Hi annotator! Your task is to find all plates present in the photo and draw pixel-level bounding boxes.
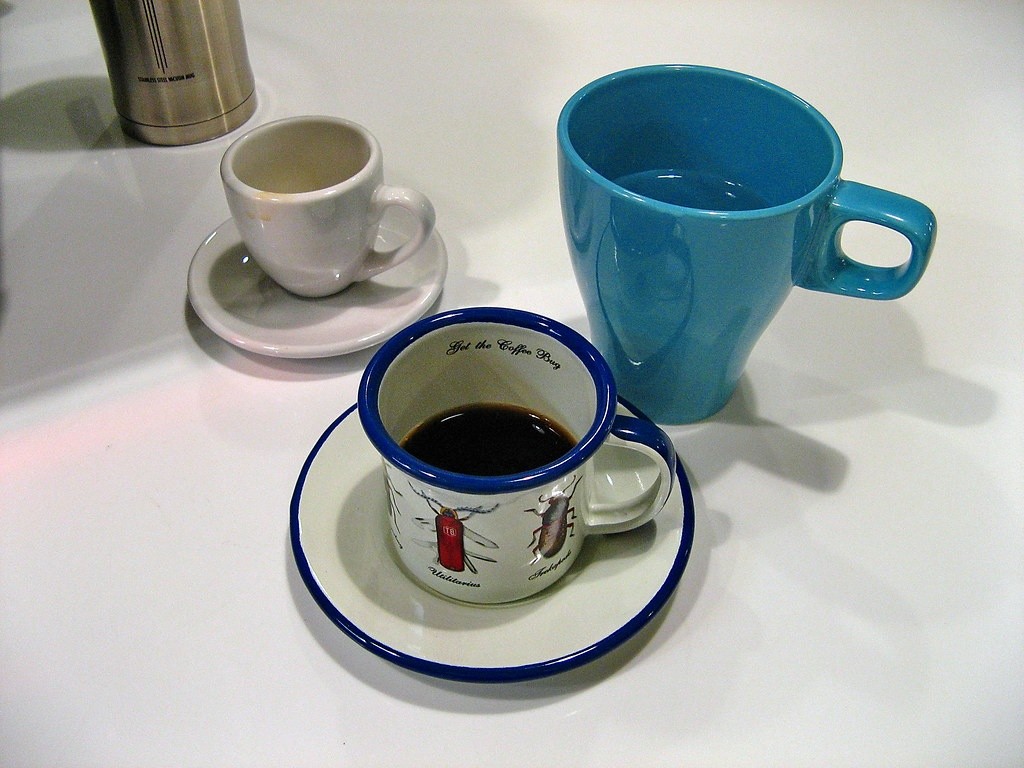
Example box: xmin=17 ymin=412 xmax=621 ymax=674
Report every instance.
xmin=288 ymin=393 xmax=695 ymax=683
xmin=188 ymin=216 xmax=448 ymax=359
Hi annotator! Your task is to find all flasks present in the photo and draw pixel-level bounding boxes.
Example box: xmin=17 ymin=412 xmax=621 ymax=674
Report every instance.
xmin=89 ymin=0 xmax=257 ymax=146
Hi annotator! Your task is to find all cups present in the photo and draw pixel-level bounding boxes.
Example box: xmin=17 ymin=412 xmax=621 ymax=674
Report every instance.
xmin=558 ymin=63 xmax=937 ymax=424
xmin=221 ymin=115 xmax=434 ymax=297
xmin=356 ymin=306 xmax=676 ymax=605
